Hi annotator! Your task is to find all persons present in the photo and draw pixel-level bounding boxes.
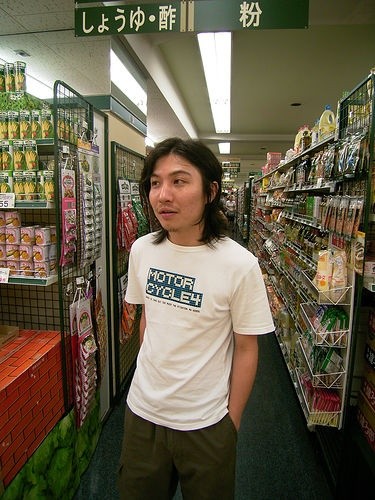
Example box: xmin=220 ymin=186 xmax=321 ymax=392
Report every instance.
xmin=116 ymin=137 xmax=276 ymax=500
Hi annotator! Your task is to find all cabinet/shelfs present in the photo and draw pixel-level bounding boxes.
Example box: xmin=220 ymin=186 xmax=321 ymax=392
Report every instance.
xmin=0 ymin=80 xmax=375 ymax=444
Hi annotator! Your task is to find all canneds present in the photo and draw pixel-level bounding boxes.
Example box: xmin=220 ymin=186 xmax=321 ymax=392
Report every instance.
xmin=0 ymin=210 xmax=58 ymax=279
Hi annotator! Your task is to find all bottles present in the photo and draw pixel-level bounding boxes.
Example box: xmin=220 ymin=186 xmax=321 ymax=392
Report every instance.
xmin=294 ymin=105 xmax=339 ymax=155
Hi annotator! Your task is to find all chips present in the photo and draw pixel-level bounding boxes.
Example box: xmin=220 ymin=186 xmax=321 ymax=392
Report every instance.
xmin=0 ymin=61 xmax=82 ymax=202
